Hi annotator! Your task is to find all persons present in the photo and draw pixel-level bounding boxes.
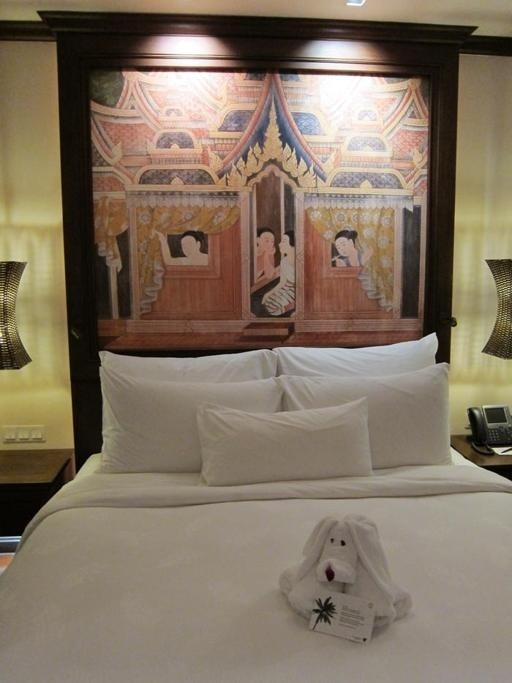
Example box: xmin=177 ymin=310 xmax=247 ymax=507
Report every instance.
xmin=262 ymin=231 xmax=295 ymax=316
xmin=154 ymin=230 xmax=209 ymax=267
xmin=334 ymin=230 xmax=362 ymax=267
xmin=256 ymin=228 xmax=276 ymax=280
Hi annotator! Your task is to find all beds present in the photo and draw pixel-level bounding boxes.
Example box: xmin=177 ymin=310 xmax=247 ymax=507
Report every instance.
xmin=0 ymin=444 xmax=511 ymax=683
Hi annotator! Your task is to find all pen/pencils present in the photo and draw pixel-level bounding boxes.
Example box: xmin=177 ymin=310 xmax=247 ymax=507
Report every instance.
xmin=501 ymin=448 xmax=512 ymax=453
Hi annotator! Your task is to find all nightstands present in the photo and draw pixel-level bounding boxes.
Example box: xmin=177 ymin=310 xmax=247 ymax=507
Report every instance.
xmin=0 ymin=448 xmax=72 ymax=531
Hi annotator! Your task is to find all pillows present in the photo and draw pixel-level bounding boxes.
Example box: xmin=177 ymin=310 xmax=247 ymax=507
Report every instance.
xmin=279 ymin=361 xmax=452 ymax=470
xmin=100 ymin=363 xmax=285 ymax=473
xmin=196 ymin=394 xmax=375 ymax=485
xmin=98 ymin=349 xmax=278 ymax=384
xmin=271 ymin=332 xmax=439 ymax=376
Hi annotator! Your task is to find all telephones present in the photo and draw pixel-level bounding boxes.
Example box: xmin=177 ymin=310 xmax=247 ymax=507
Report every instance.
xmin=467 ymin=404 xmax=512 ymax=445
